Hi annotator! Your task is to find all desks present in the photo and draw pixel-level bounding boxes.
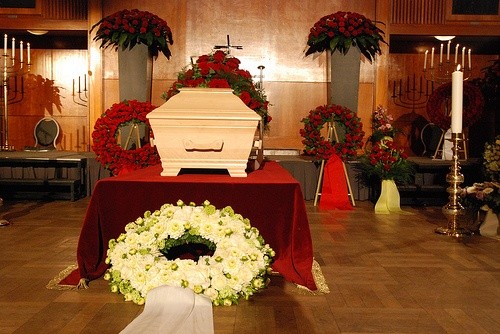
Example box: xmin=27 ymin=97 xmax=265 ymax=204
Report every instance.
xmin=408 ymin=157 xmax=471 ymax=169
xmin=46 ymin=160 xmax=317 ymax=301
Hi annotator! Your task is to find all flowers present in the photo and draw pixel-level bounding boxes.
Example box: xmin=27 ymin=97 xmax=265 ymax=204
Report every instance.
xmin=298 ymin=79 xmax=500 ymax=205
xmin=104 ymin=201 xmax=275 ymax=307
xmin=88 ymin=9 xmax=174 ymax=62
xmin=304 ymin=10 xmax=388 ymax=59
xmin=459 ymin=136 xmax=500 ymax=212
xmin=92 ymin=57 xmax=270 ymax=174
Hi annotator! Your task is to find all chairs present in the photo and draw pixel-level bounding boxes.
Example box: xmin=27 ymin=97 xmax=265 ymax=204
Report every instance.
xmin=421 ymin=123 xmax=444 ymax=154
xmin=34 ymin=118 xmax=61 ymax=149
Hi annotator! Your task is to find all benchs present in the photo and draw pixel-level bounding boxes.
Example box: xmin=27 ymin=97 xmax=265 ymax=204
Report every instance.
xmin=377 ymin=168 xmax=485 ymax=206
xmin=0 ymin=159 xmax=83 ymax=202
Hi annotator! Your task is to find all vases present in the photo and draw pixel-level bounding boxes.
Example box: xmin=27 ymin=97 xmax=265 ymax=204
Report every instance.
xmin=118 ymin=36 xmax=148 ymax=147
xmin=327 ymin=38 xmax=363 ymax=113
xmin=475 ymin=205 xmax=498 ymax=237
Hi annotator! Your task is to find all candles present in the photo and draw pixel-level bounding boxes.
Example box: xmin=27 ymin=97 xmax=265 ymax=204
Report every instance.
xmin=11 ymin=38 xmax=16 ymax=59
xmin=20 ymin=40 xmax=23 ymax=62
xmin=452 ymin=64 xmax=463 ymax=133
xmin=423 ymin=40 xmax=472 ymax=70
xmin=27 ymin=43 xmax=31 ymax=64
xmin=4 ymin=34 xmax=8 ymax=53
